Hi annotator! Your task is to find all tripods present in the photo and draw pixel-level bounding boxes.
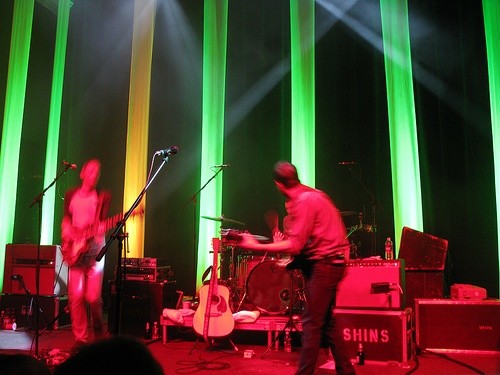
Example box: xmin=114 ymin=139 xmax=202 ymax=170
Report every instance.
xmin=262 ymin=259 xmax=303 ymax=357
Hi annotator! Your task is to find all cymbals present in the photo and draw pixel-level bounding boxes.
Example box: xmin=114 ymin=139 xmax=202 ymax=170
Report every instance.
xmin=201 ymin=216 xmax=246 ymax=226
xmin=336 ymin=210 xmax=357 ymax=217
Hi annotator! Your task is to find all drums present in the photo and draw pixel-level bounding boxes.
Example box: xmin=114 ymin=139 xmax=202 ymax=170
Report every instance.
xmin=236 ymin=254 xmax=306 ymax=314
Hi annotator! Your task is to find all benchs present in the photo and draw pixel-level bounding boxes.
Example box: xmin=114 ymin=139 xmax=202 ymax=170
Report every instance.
xmin=160 ymin=313 xmax=308 ymax=348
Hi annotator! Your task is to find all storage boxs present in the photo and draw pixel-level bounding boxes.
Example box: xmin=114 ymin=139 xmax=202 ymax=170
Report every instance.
xmin=331 ymin=309 xmax=414 ymax=365
xmin=336 ymin=259 xmax=401 ymax=309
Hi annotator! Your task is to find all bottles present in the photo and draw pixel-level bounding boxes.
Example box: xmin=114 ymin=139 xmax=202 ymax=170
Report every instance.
xmin=385 ymin=237 xmax=394 ymax=261
xmin=356 ymin=343 xmax=364 ymax=365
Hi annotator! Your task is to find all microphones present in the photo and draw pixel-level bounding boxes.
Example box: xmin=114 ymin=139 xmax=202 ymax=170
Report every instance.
xmin=155 ymin=146 xmax=179 ymax=157
xmin=372 ymin=282 xmax=397 ymax=289
xmin=338 ymin=161 xmax=356 ymax=164
xmin=64 ymin=162 xmax=77 ymax=169
xmin=213 ymin=164 xmax=232 ymax=168
xmin=11 ymin=275 xmax=19 ymax=280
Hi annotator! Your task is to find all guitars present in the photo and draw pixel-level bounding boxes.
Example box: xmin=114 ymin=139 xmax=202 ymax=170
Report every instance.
xmin=192 ymin=237 xmax=235 ymax=339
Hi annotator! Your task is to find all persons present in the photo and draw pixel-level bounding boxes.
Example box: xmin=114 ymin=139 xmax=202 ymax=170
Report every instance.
xmin=240 ymin=161 xmax=356 ymax=375
xmin=60 ymin=160 xmax=109 ymax=350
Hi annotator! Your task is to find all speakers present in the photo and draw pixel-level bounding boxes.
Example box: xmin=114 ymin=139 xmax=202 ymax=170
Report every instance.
xmin=335 ymin=227 xmax=448 ymax=310
xmin=117 ymin=281 xmax=171 ymax=337
